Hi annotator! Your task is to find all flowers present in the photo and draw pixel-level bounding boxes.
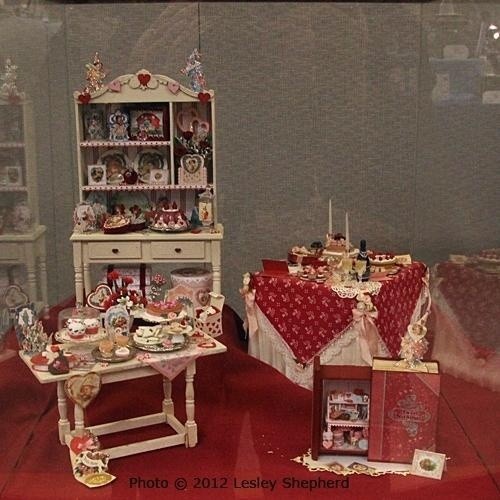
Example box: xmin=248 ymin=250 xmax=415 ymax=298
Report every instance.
xmin=104 ymin=272 xmax=147 ymax=312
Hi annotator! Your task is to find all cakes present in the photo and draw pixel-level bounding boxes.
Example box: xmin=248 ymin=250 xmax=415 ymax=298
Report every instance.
xmin=369 ymin=253 xmax=397 ymax=274
xmin=300 ymin=270 xmax=311 ymax=280
xmin=305 ymin=264 xmax=330 ymax=276
xmin=143 ymin=301 xmax=187 ymax=326
xmin=68 ymin=323 xmax=87 ymax=340
xmin=116 ymin=336 xmax=130 ymax=347
xmin=477 ymin=248 xmax=500 ymax=274
xmin=323 ymin=234 xmax=359 ymax=259
xmin=148 ymin=201 xmax=191 ymax=233
xmin=100 ymin=341 xmax=115 ymax=358
xmin=114 ymin=347 xmax=131 ymax=359
xmin=133 ymin=321 xmax=192 ymax=351
xmin=84 ymin=318 xmax=100 ymax=335
xmin=67 ymin=318 xmax=84 ymax=324
xmin=316 ymin=272 xmax=328 ymax=283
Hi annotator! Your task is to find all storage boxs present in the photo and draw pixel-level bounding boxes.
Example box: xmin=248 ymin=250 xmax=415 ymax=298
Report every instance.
xmin=311 ymin=354 xmax=440 ymax=464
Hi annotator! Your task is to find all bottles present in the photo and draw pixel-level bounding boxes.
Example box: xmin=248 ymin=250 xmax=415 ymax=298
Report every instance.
xmin=356 ymin=240 xmax=371 ymax=282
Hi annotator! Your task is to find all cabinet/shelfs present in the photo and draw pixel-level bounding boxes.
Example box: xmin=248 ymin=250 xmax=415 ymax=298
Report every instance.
xmin=0 ymin=54 xmax=51 ymax=328
xmin=72 ymin=48 xmax=225 ymax=323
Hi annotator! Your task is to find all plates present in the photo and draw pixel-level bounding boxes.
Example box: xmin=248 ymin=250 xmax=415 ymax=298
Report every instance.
xmin=132 ymin=336 xmax=189 ymax=353
xmin=91 ymin=345 xmax=136 ymax=362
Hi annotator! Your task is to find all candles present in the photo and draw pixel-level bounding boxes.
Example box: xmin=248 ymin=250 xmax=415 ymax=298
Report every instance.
xmin=327 ymin=198 xmax=333 ymax=237
xmin=345 ymin=212 xmax=350 ymax=252
